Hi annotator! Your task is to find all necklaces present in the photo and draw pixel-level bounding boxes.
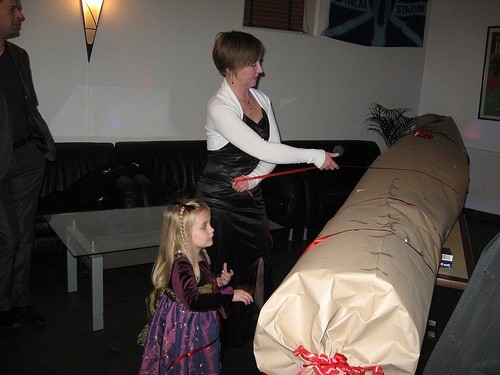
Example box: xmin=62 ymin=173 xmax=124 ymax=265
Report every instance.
xmin=238 ymin=97 xmax=254 ymax=111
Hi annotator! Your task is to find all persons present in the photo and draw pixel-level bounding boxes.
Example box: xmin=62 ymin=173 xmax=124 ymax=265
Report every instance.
xmin=0 ymin=0 xmax=58 ymax=330
xmin=138 ymin=199 xmax=255 ymax=375
xmin=192 ymin=30 xmax=341 ymax=350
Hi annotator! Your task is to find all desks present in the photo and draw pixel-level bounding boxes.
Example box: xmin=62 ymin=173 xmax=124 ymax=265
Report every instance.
xmin=41 ymin=205 xmax=285 ymax=331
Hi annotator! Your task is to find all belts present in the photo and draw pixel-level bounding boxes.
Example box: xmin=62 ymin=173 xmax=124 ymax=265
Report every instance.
xmin=14 ymin=134 xmax=33 ymax=149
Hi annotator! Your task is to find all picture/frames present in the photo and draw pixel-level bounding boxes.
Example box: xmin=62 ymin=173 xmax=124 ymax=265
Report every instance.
xmin=478 ymin=26 xmax=500 ymax=121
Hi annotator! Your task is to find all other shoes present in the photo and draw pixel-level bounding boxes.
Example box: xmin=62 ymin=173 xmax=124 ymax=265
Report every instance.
xmin=0 ymin=308 xmax=26 ymax=330
xmin=21 ymin=306 xmax=46 ymax=327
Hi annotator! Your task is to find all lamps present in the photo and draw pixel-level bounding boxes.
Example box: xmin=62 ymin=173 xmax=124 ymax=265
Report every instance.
xmin=81 ymin=0 xmax=105 ymax=63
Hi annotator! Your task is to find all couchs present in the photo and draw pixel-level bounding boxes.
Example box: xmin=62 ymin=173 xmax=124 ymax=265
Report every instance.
xmin=34 ymin=139 xmax=381 ymax=252
